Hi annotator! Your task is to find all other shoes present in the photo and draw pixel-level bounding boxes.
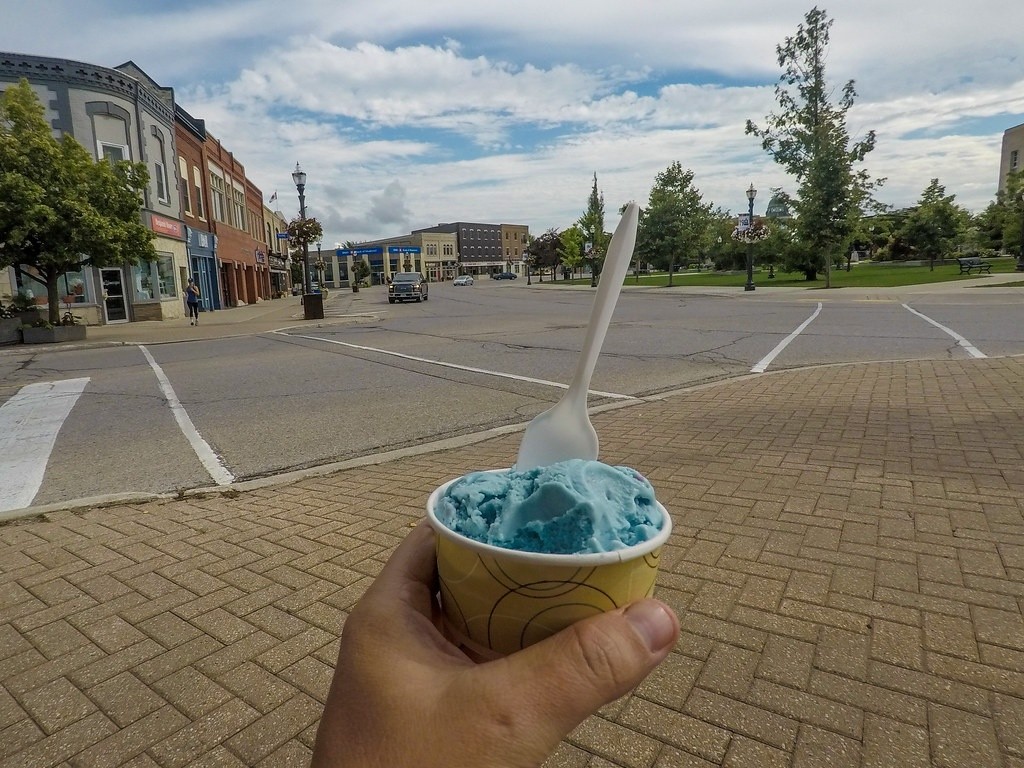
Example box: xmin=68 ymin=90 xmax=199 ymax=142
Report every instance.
xmin=191 ymin=320 xmax=194 ymax=325
xmin=195 ymin=319 xmax=200 ymax=326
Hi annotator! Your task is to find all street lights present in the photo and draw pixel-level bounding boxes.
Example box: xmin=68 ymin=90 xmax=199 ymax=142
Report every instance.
xmin=405 ymin=251 xmax=410 ymax=260
xmin=353 ymin=251 xmax=357 ymax=285
xmin=526 ymin=240 xmax=531 ymax=285
xmin=744 ymin=181 xmax=757 ymax=291
xmin=316 ymin=241 xmax=323 ymax=288
xmin=292 ymin=159 xmax=312 ymax=294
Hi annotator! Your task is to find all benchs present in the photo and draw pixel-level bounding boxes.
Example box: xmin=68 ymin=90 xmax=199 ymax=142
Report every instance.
xmin=956 ymin=257 xmax=993 ymax=276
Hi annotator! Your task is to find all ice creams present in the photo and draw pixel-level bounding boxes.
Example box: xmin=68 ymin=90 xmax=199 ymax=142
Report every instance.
xmin=425 ymin=459 xmax=672 ymax=660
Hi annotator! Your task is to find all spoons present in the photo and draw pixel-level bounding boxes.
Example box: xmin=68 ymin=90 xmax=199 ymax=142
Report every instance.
xmin=516 ymin=201 xmax=640 ymax=474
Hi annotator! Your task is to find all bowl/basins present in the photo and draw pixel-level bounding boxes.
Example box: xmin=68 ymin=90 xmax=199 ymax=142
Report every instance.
xmin=427 ymin=468 xmax=672 ymax=664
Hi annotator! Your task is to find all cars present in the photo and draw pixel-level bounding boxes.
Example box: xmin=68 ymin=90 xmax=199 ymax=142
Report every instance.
xmin=453 ymin=275 xmax=474 ymax=287
xmin=493 ymin=272 xmax=517 ymax=281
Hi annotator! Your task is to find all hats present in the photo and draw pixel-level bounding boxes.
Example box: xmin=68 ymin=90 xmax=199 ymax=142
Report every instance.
xmin=188 ymin=278 xmax=194 ymax=283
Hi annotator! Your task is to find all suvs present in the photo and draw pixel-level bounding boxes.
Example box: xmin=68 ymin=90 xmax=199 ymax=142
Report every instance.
xmin=387 ymin=272 xmax=428 ymax=304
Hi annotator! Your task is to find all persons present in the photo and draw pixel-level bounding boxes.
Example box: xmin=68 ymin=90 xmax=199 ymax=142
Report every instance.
xmin=186 ymin=278 xmax=199 ymax=326
xmin=309 ymin=518 xmax=681 ymax=768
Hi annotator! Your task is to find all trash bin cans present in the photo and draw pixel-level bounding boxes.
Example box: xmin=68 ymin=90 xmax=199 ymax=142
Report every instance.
xmin=303 ymin=293 xmax=324 ymax=320
xmin=353 ymin=286 xmax=357 ymax=292
